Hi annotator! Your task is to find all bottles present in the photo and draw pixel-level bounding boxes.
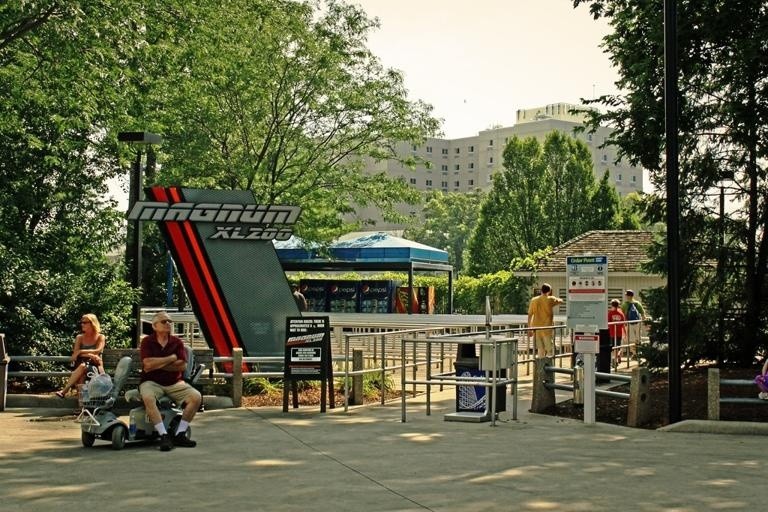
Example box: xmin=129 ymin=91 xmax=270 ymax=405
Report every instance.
xmin=82 ymin=380 xmax=91 ymax=402
xmin=129 ymin=416 xmax=137 ymax=437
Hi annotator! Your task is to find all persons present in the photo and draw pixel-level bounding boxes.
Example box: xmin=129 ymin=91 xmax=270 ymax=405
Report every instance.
xmin=621 ymin=289 xmax=647 ymax=361
xmin=137 ymin=310 xmax=202 ymax=451
xmin=289 ymin=283 xmax=308 ymax=311
xmin=54 ymin=312 xmax=106 ymax=418
xmin=608 ymin=297 xmax=628 ymax=368
xmin=527 ymin=282 xmax=564 ymax=358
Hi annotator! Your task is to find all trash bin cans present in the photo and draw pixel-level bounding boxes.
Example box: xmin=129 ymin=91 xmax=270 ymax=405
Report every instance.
xmin=572 ymin=329 xmax=613 ymax=385
xmin=0 ymin=333 xmax=11 ymax=412
xmin=454 ymin=343 xmax=506 ymax=413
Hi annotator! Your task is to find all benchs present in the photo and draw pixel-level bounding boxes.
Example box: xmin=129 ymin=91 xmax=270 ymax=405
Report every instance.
xmin=102 ymin=348 xmax=215 ymax=413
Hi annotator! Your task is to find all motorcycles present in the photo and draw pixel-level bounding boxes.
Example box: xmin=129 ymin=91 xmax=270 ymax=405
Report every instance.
xmin=78 ymin=359 xmax=205 ymax=451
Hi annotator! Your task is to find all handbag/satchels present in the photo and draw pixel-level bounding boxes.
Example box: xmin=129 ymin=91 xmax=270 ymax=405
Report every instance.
xmin=70 ymin=356 xmax=97 ymax=371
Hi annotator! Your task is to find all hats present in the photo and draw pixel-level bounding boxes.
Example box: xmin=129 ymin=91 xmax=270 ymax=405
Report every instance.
xmin=624 ymin=290 xmax=634 ymax=296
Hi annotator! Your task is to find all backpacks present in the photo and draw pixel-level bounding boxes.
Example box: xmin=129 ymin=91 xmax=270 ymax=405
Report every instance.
xmin=627 ymin=303 xmax=640 ymax=324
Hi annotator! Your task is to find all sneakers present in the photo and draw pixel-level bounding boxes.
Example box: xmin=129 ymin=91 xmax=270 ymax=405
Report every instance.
xmin=630 ymin=350 xmax=637 ymax=360
xmin=174 ymin=432 xmax=196 ymax=447
xmin=612 ymin=358 xmax=616 ymax=368
xmin=159 ymin=433 xmax=174 ymax=450
xmin=618 ymin=354 xmax=621 ymax=364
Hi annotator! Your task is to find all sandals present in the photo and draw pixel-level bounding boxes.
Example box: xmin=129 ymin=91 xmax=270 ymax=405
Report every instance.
xmin=56 ymin=391 xmax=65 ymax=398
